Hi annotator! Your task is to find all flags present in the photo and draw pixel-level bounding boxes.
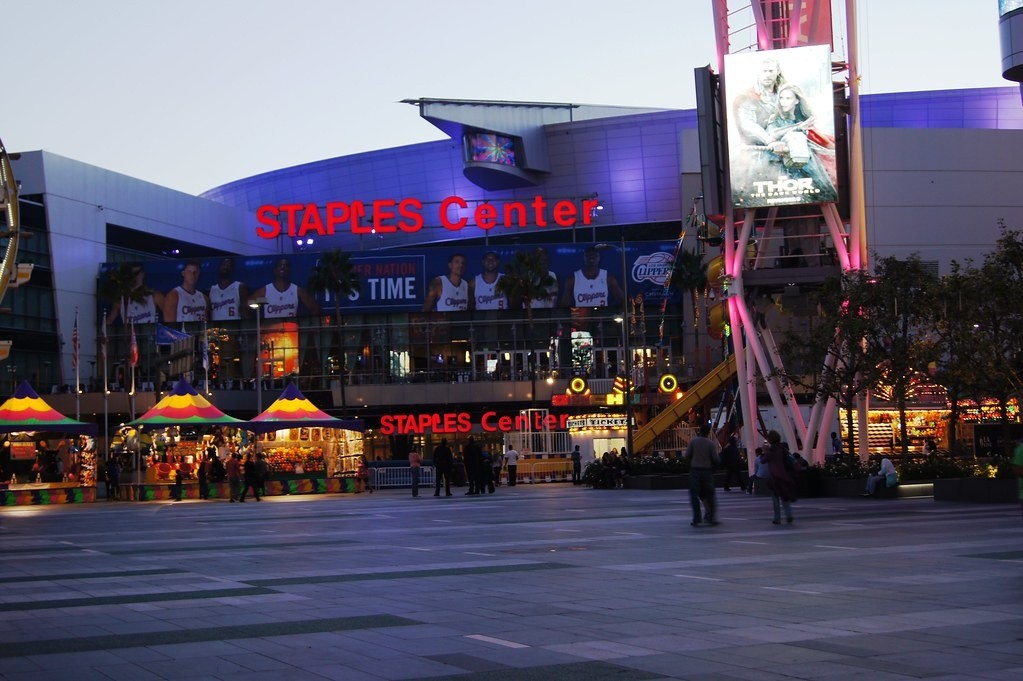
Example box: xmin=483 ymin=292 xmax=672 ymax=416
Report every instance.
xmin=72 ymin=315 xmax=81 ymax=369
xmin=203 ymin=330 xmax=208 ymax=368
xmin=100 ymin=320 xmax=138 ymax=366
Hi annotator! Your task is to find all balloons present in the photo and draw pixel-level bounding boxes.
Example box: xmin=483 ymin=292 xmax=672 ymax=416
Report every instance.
xmin=266 ymin=446 xmax=325 ymax=472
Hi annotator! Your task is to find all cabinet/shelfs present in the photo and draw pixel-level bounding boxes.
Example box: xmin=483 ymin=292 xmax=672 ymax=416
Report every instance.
xmin=892 ymin=426 xmax=943 ymax=457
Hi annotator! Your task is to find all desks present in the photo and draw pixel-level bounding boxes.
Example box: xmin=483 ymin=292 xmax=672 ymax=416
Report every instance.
xmin=408 ymin=371 xmax=457 ymax=384
xmin=51 ymin=377 xmax=267 ymax=395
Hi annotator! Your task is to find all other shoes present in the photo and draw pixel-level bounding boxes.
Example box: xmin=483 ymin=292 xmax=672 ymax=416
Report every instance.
xmin=704 ymin=519 xmax=718 ymax=525
xmin=465 ymin=491 xmax=495 ymax=497
xmin=787 ymin=517 xmax=792 ymax=523
xmin=446 ymin=494 xmax=452 ymax=496
xmin=434 ymin=494 xmax=440 ymax=496
xmin=772 ymin=520 xmax=780 ymax=524
xmin=859 ymin=491 xmax=870 ymax=496
xmin=690 ymin=521 xmax=700 ymax=525
xmin=413 ymin=495 xmax=422 ymax=498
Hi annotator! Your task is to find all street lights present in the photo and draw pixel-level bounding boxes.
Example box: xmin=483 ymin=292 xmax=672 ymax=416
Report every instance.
xmin=594 ymin=234 xmax=634 ymax=474
xmin=247 ymin=295 xmax=270 ymax=462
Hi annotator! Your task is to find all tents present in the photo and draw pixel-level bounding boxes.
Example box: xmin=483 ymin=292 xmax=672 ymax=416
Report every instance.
xmin=118 ymin=376 xmax=251 ymax=500
xmin=247 ymin=379 xmax=366 ymax=498
xmin=0 ymin=380 xmax=99 ymax=505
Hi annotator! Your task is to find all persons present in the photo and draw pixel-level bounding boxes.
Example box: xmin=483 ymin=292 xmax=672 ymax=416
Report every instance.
xmin=602 ymin=447 xmax=627 ymax=489
xmin=175 ymin=469 xmax=184 ymax=501
xmin=860 ymin=453 xmax=897 ymax=497
xmin=732 ymin=58 xmax=837 ymax=206
xmin=210 ymin=452 xmax=268 ymax=503
xmin=355 ymin=455 xmax=373 ymax=494
xmin=409 ymin=445 xmax=422 ymax=497
xmin=684 ymin=425 xmax=810 ymax=527
xmin=923 ymin=436 xmax=937 ymax=454
xmin=108 ymin=257 xmax=323 ymax=323
xmin=197 ymin=462 xmax=209 ymax=500
xmin=421 ymin=247 xmax=625 ymax=312
xmin=831 ymin=432 xmax=842 ymax=453
xmin=432 ymin=436 xmax=519 ymax=496
xmin=376 ymin=456 xmax=382 ymax=465
xmin=571 ymin=445 xmax=582 ymax=484
